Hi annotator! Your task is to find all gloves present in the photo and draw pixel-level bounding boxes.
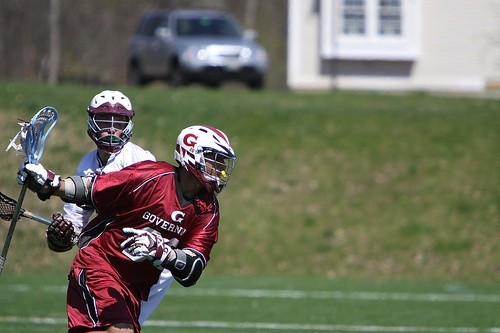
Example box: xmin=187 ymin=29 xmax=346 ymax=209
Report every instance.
xmin=16 ymin=161 xmax=61 ymax=201
xmin=46 ymin=212 xmax=74 ymax=252
xmin=121 ymin=227 xmax=171 ymax=267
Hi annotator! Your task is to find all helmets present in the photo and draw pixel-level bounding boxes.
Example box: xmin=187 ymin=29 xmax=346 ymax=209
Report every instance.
xmin=174 ymin=125 xmax=237 ymax=197
xmin=86 ymin=90 xmax=135 ymax=153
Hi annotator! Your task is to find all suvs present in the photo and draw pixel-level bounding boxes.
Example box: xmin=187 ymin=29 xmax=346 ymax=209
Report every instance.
xmin=126 ymin=6 xmax=269 ymax=91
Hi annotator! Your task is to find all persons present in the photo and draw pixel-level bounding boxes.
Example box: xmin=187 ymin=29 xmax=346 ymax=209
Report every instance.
xmin=16 ymin=125 xmax=236 ymax=333
xmin=47 ymin=91 xmax=174 ymax=325
